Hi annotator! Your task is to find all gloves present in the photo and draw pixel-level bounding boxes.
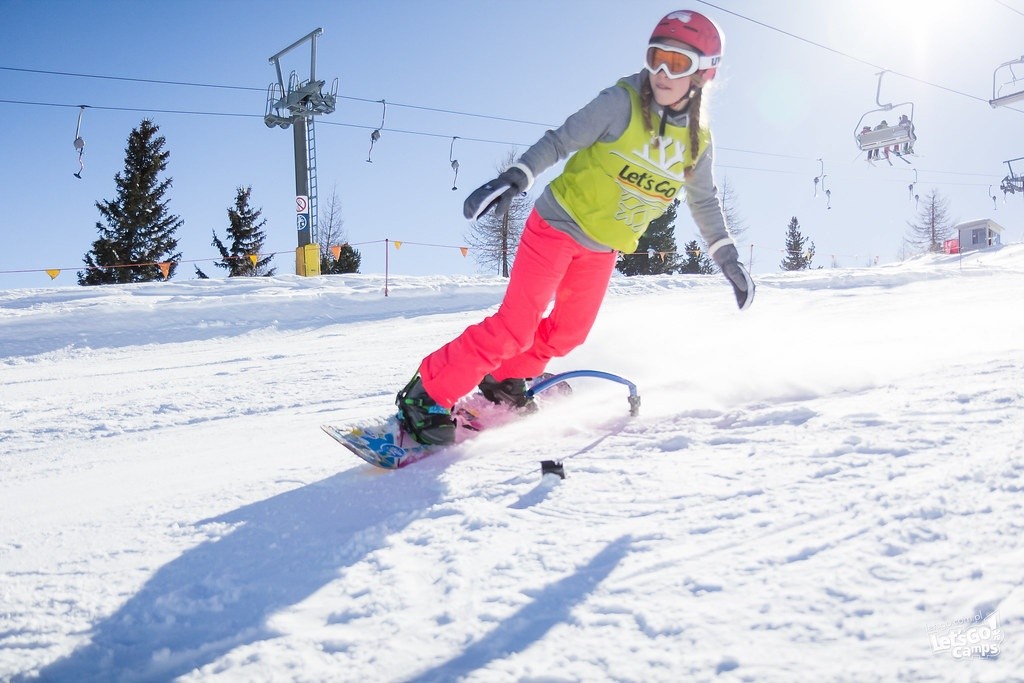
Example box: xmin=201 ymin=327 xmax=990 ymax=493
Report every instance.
xmin=709 ymin=239 xmax=756 ymax=312
xmin=462 ymin=163 xmax=535 ymax=223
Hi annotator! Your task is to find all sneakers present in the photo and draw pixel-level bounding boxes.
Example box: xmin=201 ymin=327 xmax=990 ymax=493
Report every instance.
xmin=478 ymin=373 xmax=537 ymax=416
xmin=395 ymin=371 xmax=456 ymax=446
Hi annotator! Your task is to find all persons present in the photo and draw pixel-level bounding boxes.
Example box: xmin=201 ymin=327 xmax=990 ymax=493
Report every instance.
xmin=899 ymin=115 xmax=917 ymax=154
xmin=874 ymin=120 xmax=899 ymax=158
xmin=395 ymin=11 xmax=756 ymax=447
xmin=863 ymin=126 xmax=879 ymax=161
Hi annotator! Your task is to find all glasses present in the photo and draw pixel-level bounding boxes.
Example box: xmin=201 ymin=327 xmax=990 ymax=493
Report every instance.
xmin=644 ymin=43 xmax=698 ymax=80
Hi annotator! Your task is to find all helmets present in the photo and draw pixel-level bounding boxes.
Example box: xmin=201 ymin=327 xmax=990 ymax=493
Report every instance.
xmin=649 ymin=10 xmax=722 ymax=81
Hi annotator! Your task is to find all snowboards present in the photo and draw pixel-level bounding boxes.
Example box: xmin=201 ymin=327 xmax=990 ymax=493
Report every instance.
xmin=318 ymin=372 xmax=573 ymax=470
